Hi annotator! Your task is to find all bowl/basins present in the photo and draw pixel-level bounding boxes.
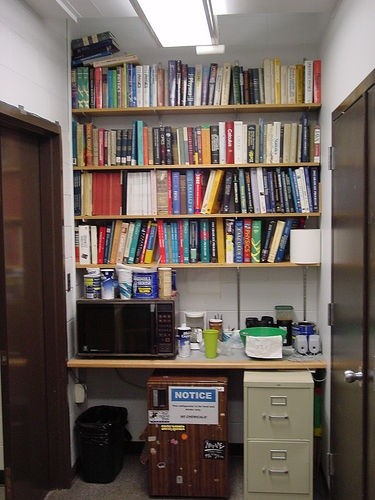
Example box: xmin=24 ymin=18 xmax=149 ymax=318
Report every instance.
xmin=239 ymin=327 xmax=288 ymax=348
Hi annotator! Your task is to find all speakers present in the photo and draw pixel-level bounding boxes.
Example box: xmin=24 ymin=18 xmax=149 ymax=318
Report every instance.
xmin=294 ymin=335 xmax=308 ymax=356
xmin=309 ymin=335 xmax=320 ymax=356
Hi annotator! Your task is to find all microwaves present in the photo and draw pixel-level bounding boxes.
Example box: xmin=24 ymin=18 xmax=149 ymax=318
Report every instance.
xmin=76 ymin=293 xmax=180 ymax=358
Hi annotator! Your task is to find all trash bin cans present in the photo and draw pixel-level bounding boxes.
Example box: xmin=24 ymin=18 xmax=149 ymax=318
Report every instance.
xmin=73 ymin=405 xmax=132 ymax=483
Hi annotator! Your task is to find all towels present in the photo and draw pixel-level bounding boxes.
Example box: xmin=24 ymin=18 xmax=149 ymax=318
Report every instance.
xmin=245 ymin=335 xmax=283 ymax=358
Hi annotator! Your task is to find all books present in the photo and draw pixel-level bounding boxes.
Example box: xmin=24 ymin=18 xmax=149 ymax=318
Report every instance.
xmin=74 ymin=167 xmax=318 ymax=216
xmin=73 ymin=118 xmax=322 ymax=166
xmin=71 ymin=31 xmax=141 ymax=67
xmin=71 ymin=56 xmax=324 ymax=109
xmin=74 ymin=217 xmax=307 ymax=264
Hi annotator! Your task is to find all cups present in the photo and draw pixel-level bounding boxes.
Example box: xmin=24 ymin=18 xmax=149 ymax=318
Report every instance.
xmin=203 ymin=330 xmax=220 ymax=358
xmin=118 ymin=269 xmax=133 ymax=300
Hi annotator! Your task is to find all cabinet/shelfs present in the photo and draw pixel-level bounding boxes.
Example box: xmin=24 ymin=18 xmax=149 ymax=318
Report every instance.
xmin=68 ymin=102 xmax=329 ymax=369
xmin=243 ymin=369 xmax=314 ymax=499
